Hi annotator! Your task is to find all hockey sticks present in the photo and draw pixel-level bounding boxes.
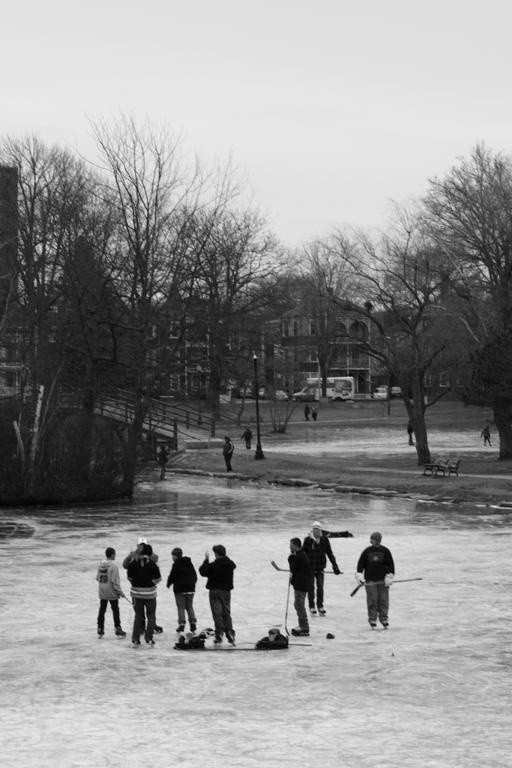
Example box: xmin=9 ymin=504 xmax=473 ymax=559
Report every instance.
xmin=351 ymin=578 xmax=421 ymax=598
xmin=285 ymin=575 xmax=290 ymax=636
xmin=271 ymin=561 xmax=343 ymax=574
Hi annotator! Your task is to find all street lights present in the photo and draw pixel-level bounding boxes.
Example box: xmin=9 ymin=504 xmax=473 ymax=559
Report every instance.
xmin=249 ymin=349 xmax=265 ymax=460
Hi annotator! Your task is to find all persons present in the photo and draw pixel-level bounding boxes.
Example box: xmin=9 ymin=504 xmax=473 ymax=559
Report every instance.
xmin=303 ymin=521 xmax=340 ymax=613
xmin=288 ymin=537 xmax=311 ymax=636
xmin=356 ymin=531 xmax=394 ymax=626
xmin=312 ymin=406 xmax=318 ymax=420
xmin=408 ymin=421 xmax=416 ymax=445
xmin=96 ymin=537 xmax=235 ymax=644
xmin=223 ymin=437 xmax=234 ymax=471
xmin=241 ymin=428 xmax=252 ymax=448
xmin=481 ymin=426 xmax=492 ymax=446
xmin=157 ymin=446 xmax=169 ymax=479
xmin=305 ymin=405 xmax=310 ymax=420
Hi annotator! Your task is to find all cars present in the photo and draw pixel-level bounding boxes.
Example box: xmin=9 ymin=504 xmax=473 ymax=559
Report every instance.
xmin=240 ymin=388 xmax=288 ymax=402
xmin=373 ymin=385 xmax=402 ymax=401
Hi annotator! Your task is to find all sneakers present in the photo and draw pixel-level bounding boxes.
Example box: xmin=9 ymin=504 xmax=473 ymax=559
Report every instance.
xmin=146 ymin=640 xmax=155 ymax=644
xmin=380 ymin=620 xmax=389 ymax=626
xmin=97 ymin=628 xmax=105 ymax=635
xmin=368 ymin=619 xmax=377 ymax=627
xmin=310 ymin=608 xmax=317 ymax=614
xmin=114 ymin=628 xmax=126 ymax=636
xmin=154 ymin=626 xmax=163 ymax=633
xmin=213 ymin=632 xmax=224 ymax=644
xmin=291 ymin=627 xmax=309 ymax=633
xmin=131 ymin=640 xmax=141 ymax=645
xmin=318 ymin=608 xmax=326 ymax=613
xmin=176 ymin=625 xmax=185 ymax=632
xmin=190 ymin=623 xmax=196 ymax=632
xmin=226 ymin=630 xmax=236 ymax=643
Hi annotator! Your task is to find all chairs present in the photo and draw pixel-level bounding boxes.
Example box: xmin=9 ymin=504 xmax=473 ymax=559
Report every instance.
xmin=434 ymin=457 xmax=460 ymax=477
xmin=423 ymin=458 xmax=449 ymax=477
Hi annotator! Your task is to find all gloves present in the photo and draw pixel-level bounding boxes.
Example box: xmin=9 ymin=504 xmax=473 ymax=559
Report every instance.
xmin=354 ymin=572 xmax=366 ymax=586
xmin=384 ymin=573 xmax=394 ymax=587
xmin=333 ymin=567 xmax=340 ymax=575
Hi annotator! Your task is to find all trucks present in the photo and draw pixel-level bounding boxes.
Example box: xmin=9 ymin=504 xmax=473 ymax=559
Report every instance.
xmin=291 ymin=376 xmax=354 ymax=402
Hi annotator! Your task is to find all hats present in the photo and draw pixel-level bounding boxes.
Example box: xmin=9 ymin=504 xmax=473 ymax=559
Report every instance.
xmin=212 ymin=544 xmax=226 ymax=556
xmin=370 ymin=532 xmax=382 ymax=546
xmin=171 ymin=547 xmax=182 ymax=556
xmin=137 ymin=537 xmax=147 ymax=545
xmin=312 ymin=520 xmax=322 ymax=529
